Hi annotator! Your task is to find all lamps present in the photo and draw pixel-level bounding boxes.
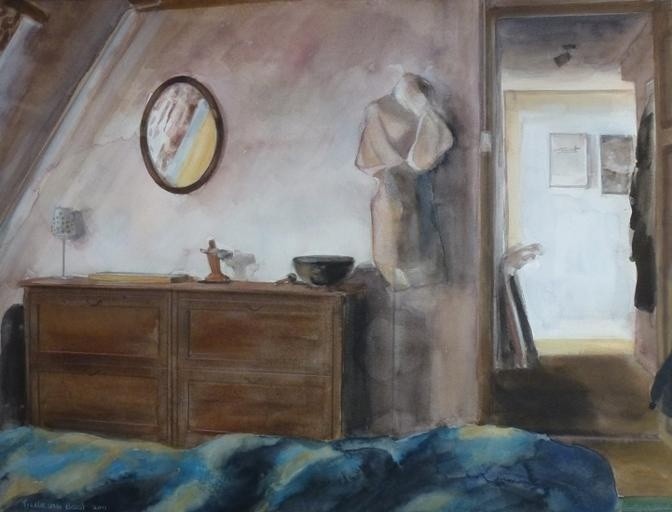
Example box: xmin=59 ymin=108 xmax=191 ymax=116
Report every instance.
xmin=51 ymin=208 xmax=76 ymax=282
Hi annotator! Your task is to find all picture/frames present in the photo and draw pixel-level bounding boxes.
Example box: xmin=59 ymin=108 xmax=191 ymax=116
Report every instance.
xmin=549 ymin=133 xmax=590 ymax=188
xmin=600 ymin=133 xmax=636 ymax=196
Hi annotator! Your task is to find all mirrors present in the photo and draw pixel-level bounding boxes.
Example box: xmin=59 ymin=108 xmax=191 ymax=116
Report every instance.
xmin=137 ymin=77 xmax=226 ymax=193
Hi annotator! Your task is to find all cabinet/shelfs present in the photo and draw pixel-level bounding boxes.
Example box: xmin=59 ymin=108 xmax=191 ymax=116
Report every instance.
xmin=20 ymin=275 xmax=173 ymax=449
xmin=173 ymin=281 xmax=365 ymax=448
